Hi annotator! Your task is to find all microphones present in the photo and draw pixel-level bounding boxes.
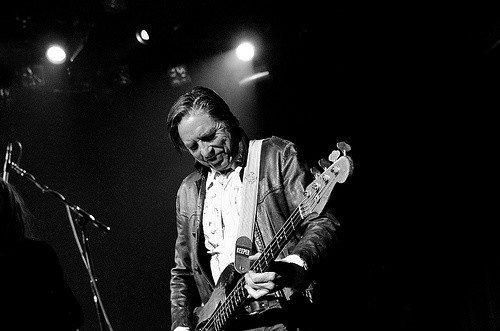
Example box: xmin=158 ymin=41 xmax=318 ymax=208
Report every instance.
xmin=3 ymin=142 xmax=12 ymax=183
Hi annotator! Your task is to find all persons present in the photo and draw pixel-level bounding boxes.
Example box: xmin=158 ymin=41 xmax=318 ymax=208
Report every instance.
xmin=168 ymin=86 xmax=342 ymax=331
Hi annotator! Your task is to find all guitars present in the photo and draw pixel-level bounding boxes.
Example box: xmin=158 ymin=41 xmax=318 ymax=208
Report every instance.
xmin=193 ymin=141 xmax=355 ymax=331
